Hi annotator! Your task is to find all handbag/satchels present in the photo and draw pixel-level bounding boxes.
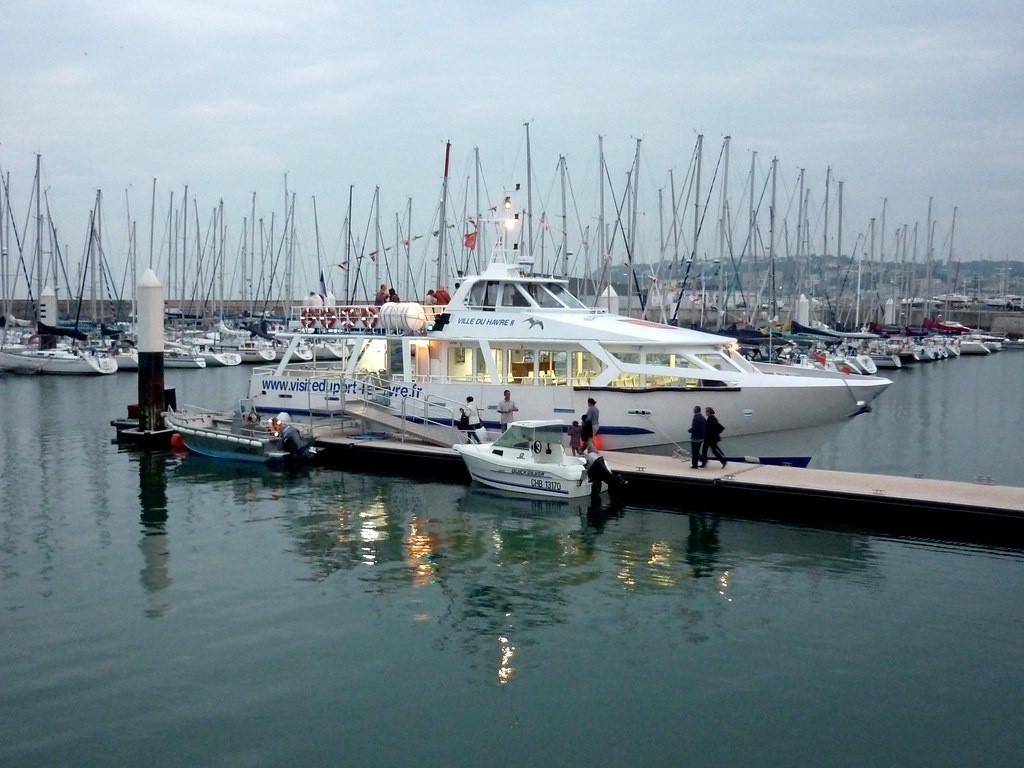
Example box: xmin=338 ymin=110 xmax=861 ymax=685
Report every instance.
xmin=717 ymin=423 xmax=725 ymax=434
xmin=474 ymin=418 xmax=482 ymax=428
xmin=457 ymin=414 xmax=469 ymax=430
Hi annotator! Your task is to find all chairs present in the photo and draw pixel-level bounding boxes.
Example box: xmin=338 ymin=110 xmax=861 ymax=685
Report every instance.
xmin=521 ymin=370 xmax=597 ymax=387
xmin=611 ymin=373 xmax=679 ymax=387
xmin=476 ymin=372 xmax=515 ymax=383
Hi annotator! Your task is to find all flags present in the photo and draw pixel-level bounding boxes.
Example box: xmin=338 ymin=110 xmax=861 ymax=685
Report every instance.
xmin=464 ymin=232 xmax=477 ymax=250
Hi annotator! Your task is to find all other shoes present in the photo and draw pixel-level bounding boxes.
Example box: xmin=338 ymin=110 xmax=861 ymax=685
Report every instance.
xmin=690 ymin=466 xmax=698 ymax=469
xmin=698 ymin=458 xmax=708 ymax=468
xmin=722 ymin=458 xmax=727 ymax=468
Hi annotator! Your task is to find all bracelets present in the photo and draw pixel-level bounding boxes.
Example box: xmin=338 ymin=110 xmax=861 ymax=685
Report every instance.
xmin=507 ymin=409 xmax=508 ymax=413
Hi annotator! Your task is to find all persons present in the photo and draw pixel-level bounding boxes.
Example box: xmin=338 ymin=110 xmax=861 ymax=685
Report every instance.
xmin=308 ymin=292 xmax=323 ymax=321
xmin=496 ymin=390 xmax=519 ymax=434
xmin=688 ymin=406 xmax=727 ymax=469
xmin=375 ymin=284 xmax=400 ymax=310
xmin=426 ymin=283 xmax=460 ymax=305
xmin=461 ymin=396 xmax=481 ymax=444
xmin=568 ymin=398 xmax=600 ymax=456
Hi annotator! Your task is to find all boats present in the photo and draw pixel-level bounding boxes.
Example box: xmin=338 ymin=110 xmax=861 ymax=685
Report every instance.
xmin=161 ymin=403 xmax=325 ymax=462
xmin=453 ymin=419 xmax=612 ymax=499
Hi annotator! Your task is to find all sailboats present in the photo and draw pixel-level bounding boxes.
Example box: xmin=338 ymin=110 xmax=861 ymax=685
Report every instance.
xmin=0 ymin=116 xmax=1023 ymax=449
